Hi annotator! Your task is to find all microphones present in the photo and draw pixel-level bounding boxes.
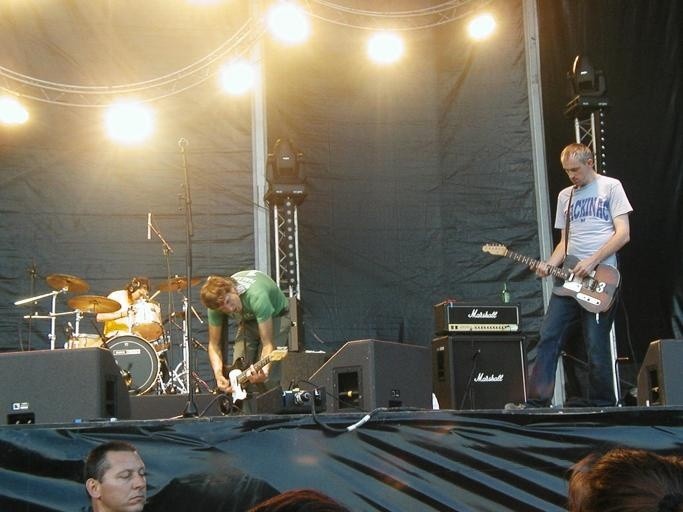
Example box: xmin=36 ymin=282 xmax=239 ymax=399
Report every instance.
xmin=174 ymin=136 xmax=196 ymax=170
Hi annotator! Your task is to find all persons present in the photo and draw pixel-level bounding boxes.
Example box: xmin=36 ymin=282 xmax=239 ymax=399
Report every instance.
xmin=564 ymin=449 xmax=683 ymax=511
xmin=96 ymin=277 xmax=175 ymax=393
xmin=200 ymin=270 xmax=292 ymax=393
xmin=80 ymin=443 xmax=147 ymax=512
xmin=503 ymin=143 xmax=631 ymax=411
xmin=247 ymin=489 xmax=346 ymax=512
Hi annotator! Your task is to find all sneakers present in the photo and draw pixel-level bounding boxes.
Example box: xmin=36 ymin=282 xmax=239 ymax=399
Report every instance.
xmin=503 ymin=400 xmax=528 ymax=412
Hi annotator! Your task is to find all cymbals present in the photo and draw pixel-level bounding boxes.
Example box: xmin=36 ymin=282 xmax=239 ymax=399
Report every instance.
xmin=68 ymin=295 xmax=121 ymax=315
xmin=159 ymin=276 xmax=200 ymax=292
xmin=46 ymin=273 xmax=90 ymax=293
xmin=171 ymin=310 xmax=201 ymax=320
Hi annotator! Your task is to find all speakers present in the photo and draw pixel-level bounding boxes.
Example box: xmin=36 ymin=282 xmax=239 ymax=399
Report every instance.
xmin=0 ymin=347 xmax=131 ymax=423
xmin=637 ymin=341 xmax=683 ymax=408
xmin=431 ymin=336 xmax=530 ymax=410
xmin=308 ymin=340 xmax=434 ymax=412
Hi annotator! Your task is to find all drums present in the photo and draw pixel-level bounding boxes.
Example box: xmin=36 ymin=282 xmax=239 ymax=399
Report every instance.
xmin=70 ymin=333 xmax=98 ymax=349
xmin=126 ymin=299 xmax=163 ymax=342
xmin=155 ymin=335 xmax=171 ymax=351
xmin=102 ymin=335 xmax=160 ymax=397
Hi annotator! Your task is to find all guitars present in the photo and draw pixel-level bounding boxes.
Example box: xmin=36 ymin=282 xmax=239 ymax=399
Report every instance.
xmin=216 ymin=346 xmax=289 ymax=415
xmin=481 ymin=242 xmax=623 ymax=315
